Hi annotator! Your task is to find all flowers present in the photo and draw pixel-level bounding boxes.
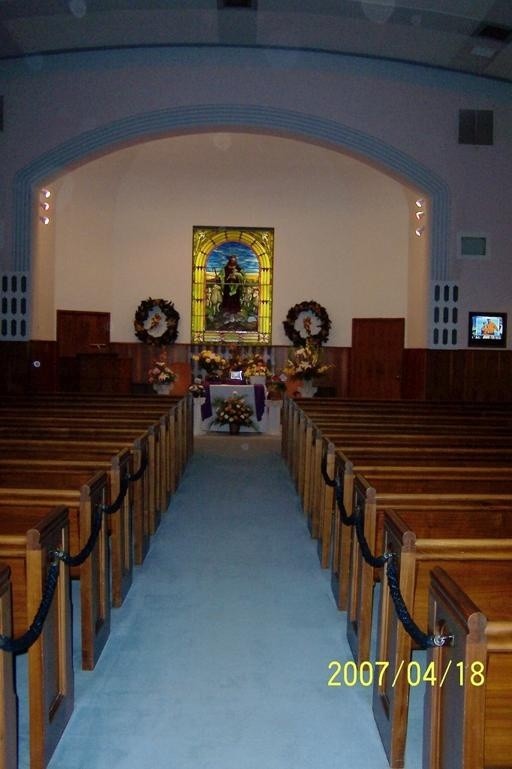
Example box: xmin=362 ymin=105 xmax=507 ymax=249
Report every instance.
xmin=188 ymin=348 xmax=280 ymax=435
xmin=149 ymin=352 xmax=177 ymax=384
xmin=280 ymin=348 xmax=334 ymax=382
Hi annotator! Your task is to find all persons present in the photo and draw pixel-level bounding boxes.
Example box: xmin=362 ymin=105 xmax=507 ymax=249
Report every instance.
xmin=480 ymin=317 xmax=499 ymax=339
xmin=215 ymin=255 xmax=246 ymax=325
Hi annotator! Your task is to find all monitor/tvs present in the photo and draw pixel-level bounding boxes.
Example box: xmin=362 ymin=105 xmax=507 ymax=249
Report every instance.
xmin=467 ymin=311 xmax=508 ymax=348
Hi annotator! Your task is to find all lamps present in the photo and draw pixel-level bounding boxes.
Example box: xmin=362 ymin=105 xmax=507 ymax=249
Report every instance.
xmin=415 ymin=199 xmax=424 ymax=209
xmin=41 ymin=201 xmax=50 ymax=212
xmin=41 ymin=189 xmax=50 ymax=199
xmin=41 ymin=215 xmax=50 ymax=225
xmin=416 ymin=212 xmax=425 ymax=221
xmin=415 ymin=226 xmax=425 ymax=237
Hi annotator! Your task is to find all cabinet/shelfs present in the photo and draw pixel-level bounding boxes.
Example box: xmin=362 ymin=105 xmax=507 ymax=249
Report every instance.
xmin=76 ymin=352 xmax=133 ymax=393
